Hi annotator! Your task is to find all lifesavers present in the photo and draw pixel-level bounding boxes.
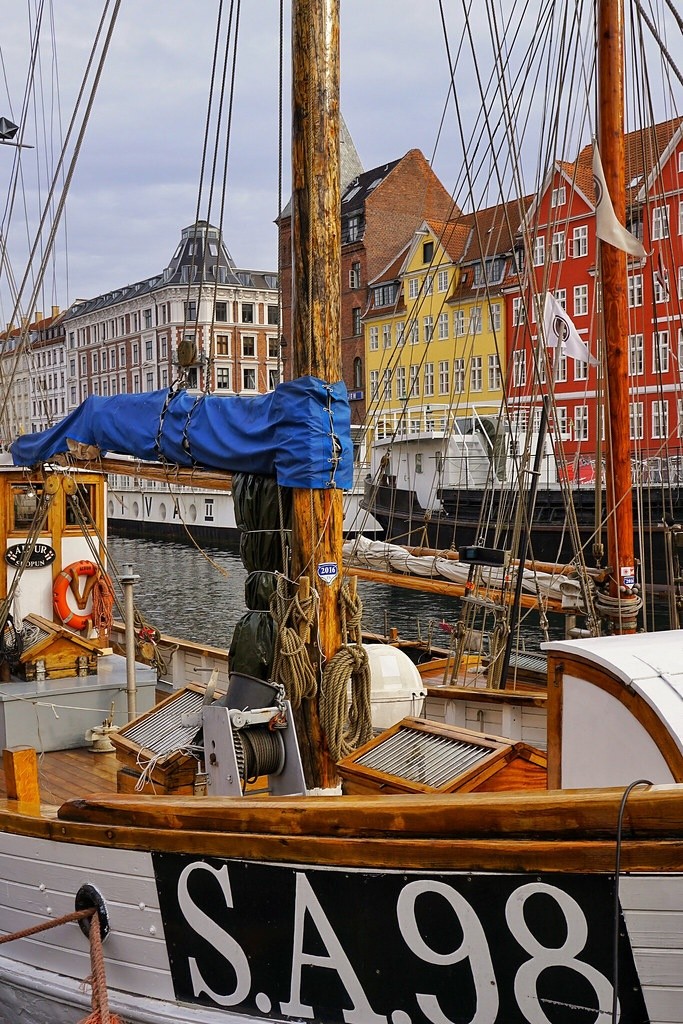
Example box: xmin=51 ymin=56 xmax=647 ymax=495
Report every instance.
xmin=53 ymin=560 xmax=114 ymax=631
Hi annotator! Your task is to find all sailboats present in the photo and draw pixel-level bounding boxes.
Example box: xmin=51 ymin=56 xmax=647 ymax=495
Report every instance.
xmin=0 ymin=0 xmax=683 ymax=1024
